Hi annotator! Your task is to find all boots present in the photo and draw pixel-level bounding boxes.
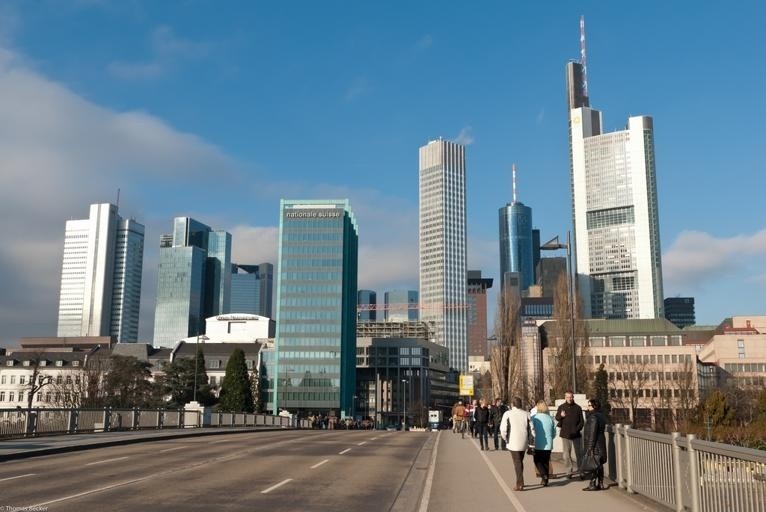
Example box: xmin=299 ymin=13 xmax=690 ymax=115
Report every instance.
xmin=583 ymin=471 xmax=597 ymax=491
xmin=596 ymin=471 xmax=604 ymax=490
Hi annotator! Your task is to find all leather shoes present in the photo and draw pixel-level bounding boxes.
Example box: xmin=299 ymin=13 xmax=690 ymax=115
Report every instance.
xmin=512 ymin=486 xmax=523 ymax=491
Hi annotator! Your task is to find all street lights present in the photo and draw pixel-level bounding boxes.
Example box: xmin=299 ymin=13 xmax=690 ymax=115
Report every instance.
xmin=353 ymin=395 xmax=368 ymax=431
xmin=486 ymin=331 xmax=509 ymax=343
xmin=193 ymin=328 xmax=210 ymax=402
xmin=402 ymin=379 xmax=407 ymax=431
xmin=540 ymin=228 xmax=575 ymax=388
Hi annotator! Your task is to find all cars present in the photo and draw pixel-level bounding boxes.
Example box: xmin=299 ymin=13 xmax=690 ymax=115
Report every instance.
xmin=427 ymin=423 xmax=449 ymax=431
xmin=384 ymin=424 xmax=400 ymax=431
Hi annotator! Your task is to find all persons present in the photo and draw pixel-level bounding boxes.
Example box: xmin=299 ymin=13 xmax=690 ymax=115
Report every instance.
xmin=555 ymin=391 xmax=585 ymax=480
xmin=530 ymin=399 xmax=557 ymax=486
xmin=295 ymin=411 xmax=302 ymax=427
xmin=451 ymin=397 xmax=536 ymax=455
xmin=529 ymin=400 xmax=554 ymax=479
xmin=500 ymin=397 xmax=535 ymax=490
xmin=581 ymin=398 xmax=607 ymax=491
xmin=308 ymin=414 xmax=375 ymax=430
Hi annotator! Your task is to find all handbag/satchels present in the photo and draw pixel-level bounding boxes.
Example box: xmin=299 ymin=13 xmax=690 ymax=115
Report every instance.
xmin=581 ymin=449 xmax=602 ymax=472
